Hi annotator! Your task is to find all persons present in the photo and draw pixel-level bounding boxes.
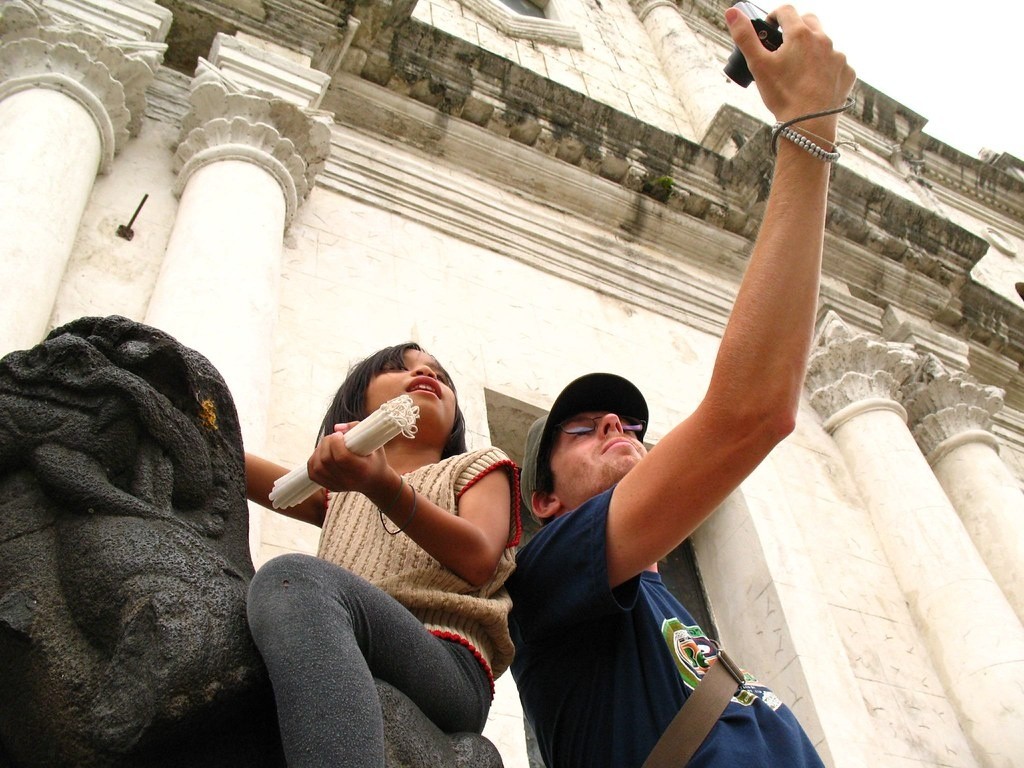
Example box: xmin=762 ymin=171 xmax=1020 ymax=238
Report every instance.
xmin=245 ymin=341 xmax=524 ymax=768
xmin=499 ymin=2 xmax=860 ymax=767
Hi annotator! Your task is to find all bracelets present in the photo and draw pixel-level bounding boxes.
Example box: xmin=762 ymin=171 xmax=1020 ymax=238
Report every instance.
xmin=378 ymin=476 xmax=417 ymax=536
xmin=772 ymin=121 xmax=841 ymax=163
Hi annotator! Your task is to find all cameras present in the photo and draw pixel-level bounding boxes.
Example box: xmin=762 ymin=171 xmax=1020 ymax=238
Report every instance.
xmin=723 ymin=2 xmax=783 ymax=88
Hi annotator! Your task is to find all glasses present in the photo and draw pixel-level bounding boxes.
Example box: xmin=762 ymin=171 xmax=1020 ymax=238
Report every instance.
xmin=547 ymin=416 xmax=647 ymax=465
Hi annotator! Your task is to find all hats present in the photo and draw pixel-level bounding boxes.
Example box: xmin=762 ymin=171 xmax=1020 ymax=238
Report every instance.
xmin=521 ymin=372 xmax=649 ymax=525
